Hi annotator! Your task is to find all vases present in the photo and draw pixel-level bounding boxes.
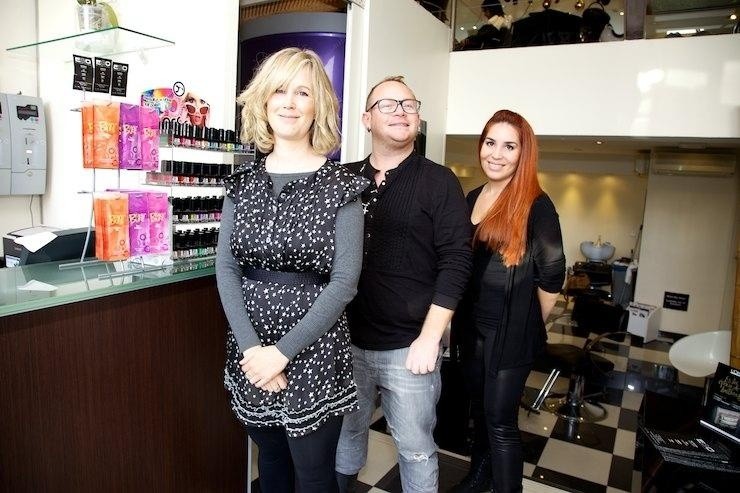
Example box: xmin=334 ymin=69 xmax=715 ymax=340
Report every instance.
xmin=78 ymin=5 xmax=102 ymax=35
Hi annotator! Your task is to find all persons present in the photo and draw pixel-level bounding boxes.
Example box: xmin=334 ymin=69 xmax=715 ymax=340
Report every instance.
xmin=446 ymin=109 xmax=567 ymax=493
xmin=214 ymin=46 xmax=372 ymax=493
xmin=459 ymin=1 xmax=512 ymax=50
xmin=330 ymin=75 xmax=472 ymax=492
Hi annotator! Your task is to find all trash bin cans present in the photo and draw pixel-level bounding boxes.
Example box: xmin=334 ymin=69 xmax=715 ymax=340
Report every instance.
xmin=611 ymin=260 xmax=637 ymax=307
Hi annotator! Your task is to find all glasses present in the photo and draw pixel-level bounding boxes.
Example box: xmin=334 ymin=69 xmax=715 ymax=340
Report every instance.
xmin=367 ymin=99 xmax=422 ymax=114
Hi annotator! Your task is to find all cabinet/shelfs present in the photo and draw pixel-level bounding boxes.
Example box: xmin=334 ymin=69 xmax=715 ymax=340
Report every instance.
xmin=141 ymin=142 xmax=257 ymax=262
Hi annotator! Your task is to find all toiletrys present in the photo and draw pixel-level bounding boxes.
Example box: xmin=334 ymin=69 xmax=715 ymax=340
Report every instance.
xmin=147 ymin=119 xmax=235 ymax=259
xmin=171 ymin=261 xmax=213 ymax=274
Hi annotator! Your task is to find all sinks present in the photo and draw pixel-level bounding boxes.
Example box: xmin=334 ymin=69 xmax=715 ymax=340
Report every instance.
xmin=580 ymin=240 xmax=616 ymax=261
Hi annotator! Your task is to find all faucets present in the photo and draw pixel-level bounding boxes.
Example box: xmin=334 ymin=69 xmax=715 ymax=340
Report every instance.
xmin=594 ymin=234 xmax=603 ymax=247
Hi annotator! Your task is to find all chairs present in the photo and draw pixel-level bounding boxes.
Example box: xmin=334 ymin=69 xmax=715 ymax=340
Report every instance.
xmin=669 ymin=329 xmax=733 ymax=406
xmin=520 ymin=330 xmax=635 ymax=423
